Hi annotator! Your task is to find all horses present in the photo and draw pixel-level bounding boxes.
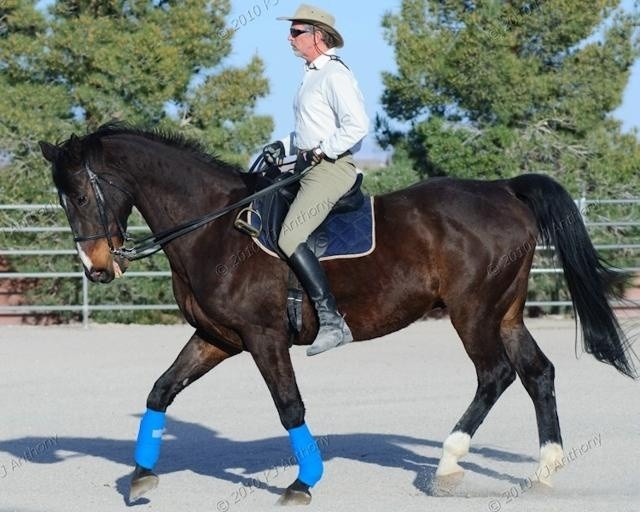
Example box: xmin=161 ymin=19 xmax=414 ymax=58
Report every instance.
xmin=38 ymin=118 xmax=637 ymax=506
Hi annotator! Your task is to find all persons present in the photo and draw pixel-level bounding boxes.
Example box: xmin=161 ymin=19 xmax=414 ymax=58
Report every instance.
xmin=263 ymin=5 xmax=371 ymax=356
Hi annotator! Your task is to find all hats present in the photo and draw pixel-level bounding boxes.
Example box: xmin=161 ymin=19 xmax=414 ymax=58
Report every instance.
xmin=276 ymin=5 xmax=343 ymax=49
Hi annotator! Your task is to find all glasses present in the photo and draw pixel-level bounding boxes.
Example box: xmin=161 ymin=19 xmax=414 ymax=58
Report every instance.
xmin=289 ymin=27 xmax=318 ymax=36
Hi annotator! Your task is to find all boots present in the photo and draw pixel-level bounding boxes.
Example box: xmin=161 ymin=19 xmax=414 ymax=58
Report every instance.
xmin=288 ymin=243 xmax=353 ymax=355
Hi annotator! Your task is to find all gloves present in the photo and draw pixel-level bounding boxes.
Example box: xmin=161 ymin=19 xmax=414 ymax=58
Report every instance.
xmin=263 ymin=141 xmax=285 ymax=167
xmin=296 ymin=150 xmax=322 ymax=175
